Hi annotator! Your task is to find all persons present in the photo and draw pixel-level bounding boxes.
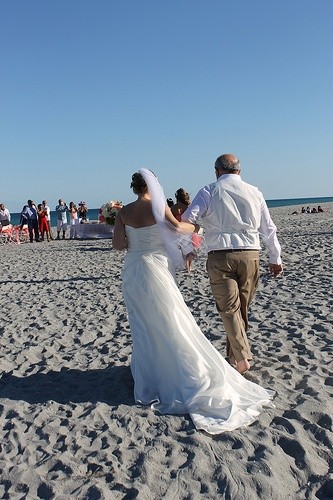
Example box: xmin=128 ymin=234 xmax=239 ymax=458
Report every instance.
xmin=180 ymin=155 xmax=283 ymax=374
xmin=69 ymin=202 xmax=79 ymax=239
xmin=20 ymin=200 xmax=42 ymax=242
xmin=302 ymin=206 xmax=323 ymax=213
xmin=55 ymin=199 xmax=68 ymax=240
xmin=42 ymin=200 xmax=54 ymax=240
xmin=0 ymin=203 xmax=10 ymax=243
xmin=167 ymin=197 xmax=174 ymax=209
xmin=171 ymin=188 xmax=202 ymax=272
xmin=37 ymin=204 xmax=50 ymax=242
xmin=112 ymin=169 xmax=276 ymax=435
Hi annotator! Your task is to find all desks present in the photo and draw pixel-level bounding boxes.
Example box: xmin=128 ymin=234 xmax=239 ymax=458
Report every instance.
xmin=73 ymin=224 xmax=115 ymax=240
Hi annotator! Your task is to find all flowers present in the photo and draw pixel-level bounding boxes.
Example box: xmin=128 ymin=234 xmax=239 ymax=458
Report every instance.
xmin=98 ymin=201 xmax=125 ymax=223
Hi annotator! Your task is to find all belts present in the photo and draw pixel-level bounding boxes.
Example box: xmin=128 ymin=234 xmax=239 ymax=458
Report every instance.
xmin=208 ymin=248 xmax=259 ymax=254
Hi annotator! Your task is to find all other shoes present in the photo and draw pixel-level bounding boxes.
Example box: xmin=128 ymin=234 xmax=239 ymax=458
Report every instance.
xmin=55 ymin=237 xmax=59 ymax=240
xmin=62 ymin=237 xmax=65 ymax=240
xmin=36 ymin=240 xmax=42 ymax=242
xmin=50 ymin=236 xmax=54 ymax=240
xmin=47 ymin=239 xmax=50 ymax=242
xmin=30 ymin=240 xmax=33 ymax=243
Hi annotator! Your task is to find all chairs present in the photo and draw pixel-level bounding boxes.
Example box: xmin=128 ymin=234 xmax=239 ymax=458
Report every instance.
xmin=0 ymin=223 xmax=28 ymax=245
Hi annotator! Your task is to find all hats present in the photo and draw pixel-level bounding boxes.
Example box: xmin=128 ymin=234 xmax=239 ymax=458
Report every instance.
xmin=79 ymin=201 xmax=85 ymax=205
xmin=28 ymin=200 xmax=33 ymax=203
